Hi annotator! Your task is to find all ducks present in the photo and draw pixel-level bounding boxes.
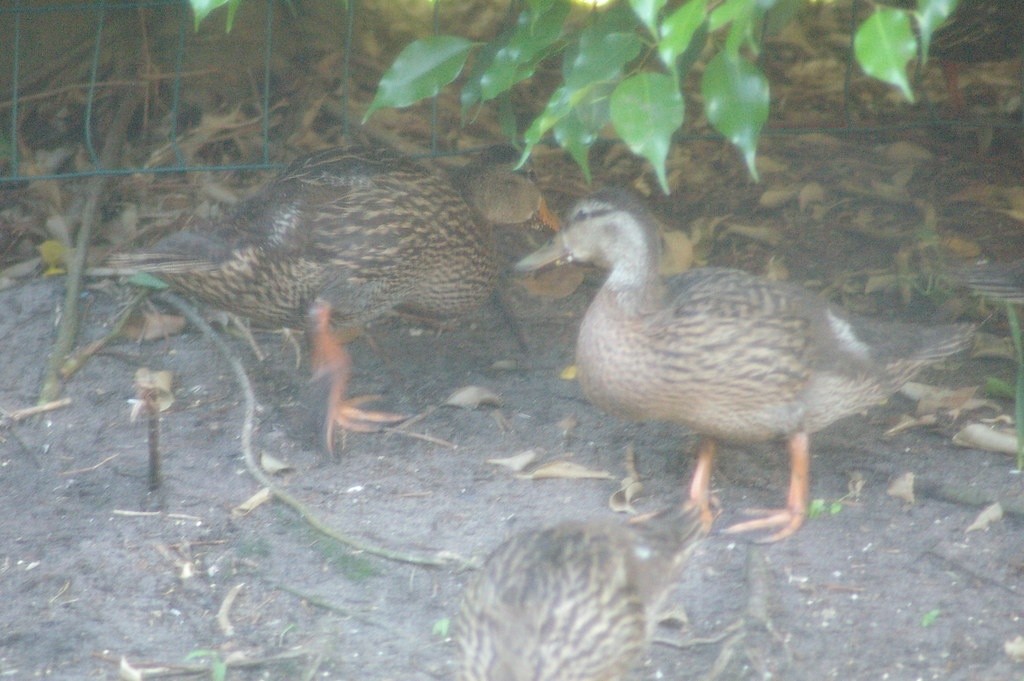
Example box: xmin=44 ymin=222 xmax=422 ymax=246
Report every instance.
xmin=98 ymin=140 xmax=562 ymax=463
xmin=511 ymin=189 xmax=994 ymax=547
